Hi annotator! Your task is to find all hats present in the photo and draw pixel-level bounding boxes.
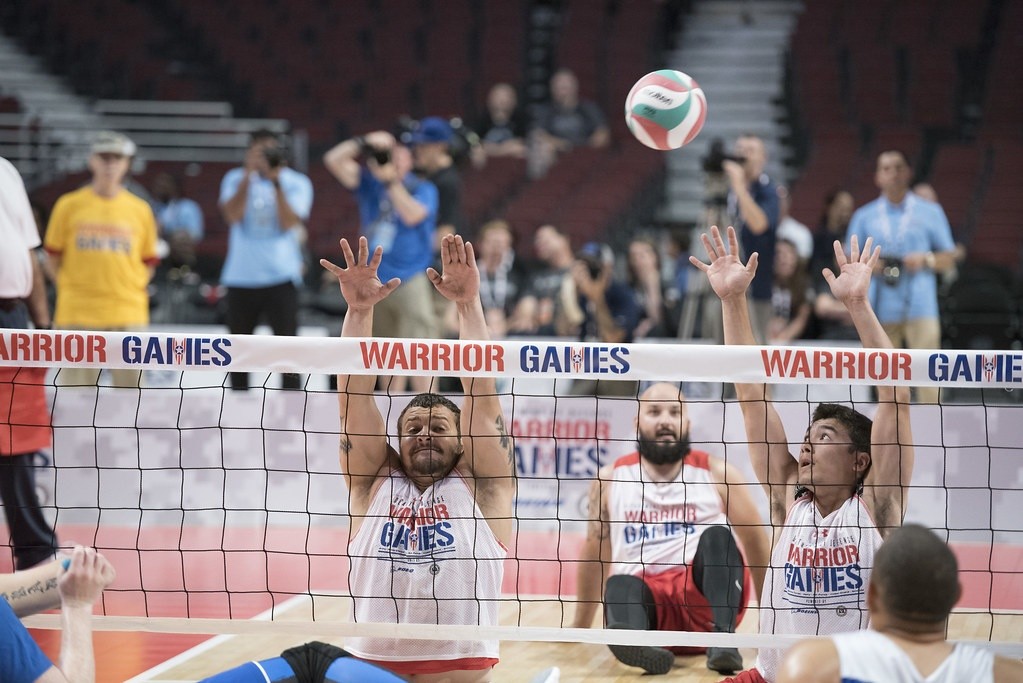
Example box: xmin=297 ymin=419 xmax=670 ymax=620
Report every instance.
xmin=92 ymin=130 xmax=135 ymax=157
xmin=402 ymin=118 xmax=451 ymax=145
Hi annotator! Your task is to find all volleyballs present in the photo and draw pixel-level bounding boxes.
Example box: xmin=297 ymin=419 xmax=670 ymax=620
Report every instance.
xmin=625 ymin=70 xmax=707 ymax=150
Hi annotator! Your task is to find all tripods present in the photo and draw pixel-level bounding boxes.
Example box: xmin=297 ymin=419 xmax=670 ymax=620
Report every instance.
xmin=680 ymin=173 xmax=763 ymax=349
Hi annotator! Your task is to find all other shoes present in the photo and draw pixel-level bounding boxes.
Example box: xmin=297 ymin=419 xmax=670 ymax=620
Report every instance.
xmin=605 ymin=623 xmax=673 ymax=675
xmin=706 ymin=647 xmax=743 ymax=672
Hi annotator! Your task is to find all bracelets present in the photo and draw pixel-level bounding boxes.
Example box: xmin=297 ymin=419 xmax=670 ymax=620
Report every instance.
xmin=353 ymin=134 xmax=372 ymax=154
xmin=925 ymin=252 xmax=936 ymax=269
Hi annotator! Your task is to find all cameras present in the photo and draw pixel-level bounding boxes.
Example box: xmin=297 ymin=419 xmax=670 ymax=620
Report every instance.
xmin=259 ymin=147 xmax=287 ymax=170
xmin=700 ymin=137 xmax=746 ymax=173
xmin=373 ymin=150 xmax=390 ymax=164
xmin=877 ymin=256 xmax=904 ymax=286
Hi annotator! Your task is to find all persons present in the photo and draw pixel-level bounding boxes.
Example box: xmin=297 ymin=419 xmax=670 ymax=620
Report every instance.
xmin=0 ymin=545 xmax=116 ymax=683
xmin=322 ymin=129 xmax=440 ymax=396
xmin=688 ymin=226 xmax=914 ymax=683
xmin=538 ymin=67 xmax=609 ymax=152
xmin=776 ymin=525 xmax=1021 ymax=683
xmin=320 ymin=232 xmax=519 ymax=683
xmin=833 ymin=150 xmax=956 ymax=406
xmin=45 ymin=131 xmax=161 ymax=392
xmin=557 ymin=383 xmax=774 ymax=673
xmin=700 ymin=133 xmax=780 ymax=402
xmin=455 ymin=81 xmax=540 ymax=159
xmin=218 ymin=130 xmax=314 ymax=394
xmin=0 ymin=61 xmax=963 ymax=588
